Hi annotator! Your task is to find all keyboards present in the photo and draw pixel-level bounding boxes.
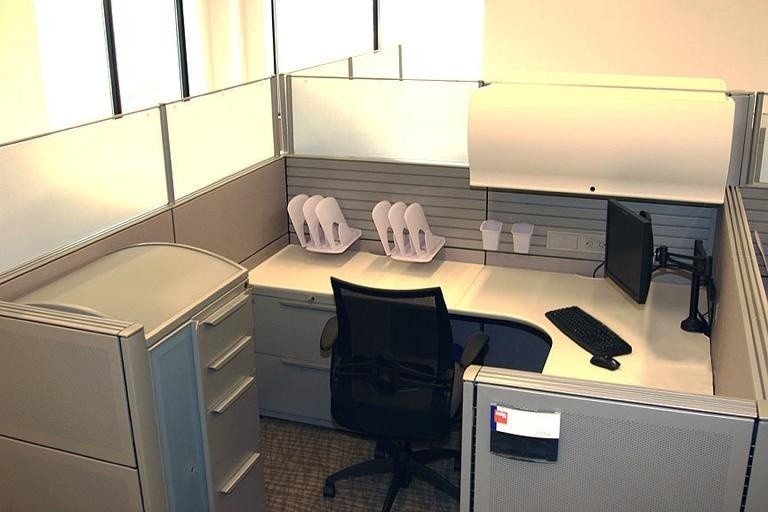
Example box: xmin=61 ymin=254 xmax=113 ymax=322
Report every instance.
xmin=545 ymin=306 xmax=632 ymax=357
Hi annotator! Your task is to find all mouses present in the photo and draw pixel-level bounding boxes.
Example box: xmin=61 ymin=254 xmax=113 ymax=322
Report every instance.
xmin=590 ymin=354 xmax=621 ymax=371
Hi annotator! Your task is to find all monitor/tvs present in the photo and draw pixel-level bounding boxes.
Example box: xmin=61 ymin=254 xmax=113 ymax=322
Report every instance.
xmin=604 ymin=199 xmax=654 ymax=304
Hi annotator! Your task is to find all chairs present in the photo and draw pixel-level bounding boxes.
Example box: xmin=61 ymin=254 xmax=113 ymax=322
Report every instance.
xmin=320 ymin=276 xmax=491 ymax=512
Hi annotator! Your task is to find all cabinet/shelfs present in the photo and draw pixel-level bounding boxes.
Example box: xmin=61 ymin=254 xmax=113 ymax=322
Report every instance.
xmin=0 ymin=242 xmax=266 ymax=512
xmin=246 ymin=243 xmax=715 ymax=512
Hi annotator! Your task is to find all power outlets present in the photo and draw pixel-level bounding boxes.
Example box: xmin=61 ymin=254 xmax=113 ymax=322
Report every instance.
xmin=580 ymin=234 xmax=606 ymax=254
